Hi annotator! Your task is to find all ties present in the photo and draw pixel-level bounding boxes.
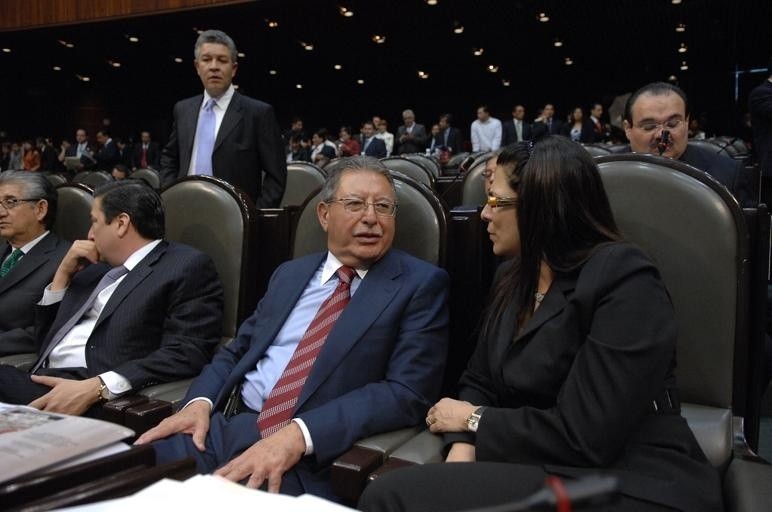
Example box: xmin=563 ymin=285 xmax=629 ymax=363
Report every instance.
xmin=546 ymin=116 xmax=551 ymax=134
xmin=30 ymin=266 xmax=129 ymax=374
xmin=142 ymin=143 xmax=146 ymax=169
xmin=257 ymin=265 xmax=357 ymax=438
xmin=79 ymin=144 xmax=82 ymax=159
xmin=0 ymin=249 xmax=24 ymax=279
xmin=195 ymin=99 xmax=216 ymax=176
xmin=516 ymin=120 xmax=521 ymax=142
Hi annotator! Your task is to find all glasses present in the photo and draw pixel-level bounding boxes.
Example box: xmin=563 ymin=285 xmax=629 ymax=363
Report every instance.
xmin=487 ymin=189 xmax=517 ymax=207
xmin=636 ymin=119 xmax=681 ymax=134
xmin=326 ymin=198 xmax=398 ymax=217
xmin=480 ymin=168 xmax=495 ymax=179
xmin=1 ymin=199 xmax=39 ymax=209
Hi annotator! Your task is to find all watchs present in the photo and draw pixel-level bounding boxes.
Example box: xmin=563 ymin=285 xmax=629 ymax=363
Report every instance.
xmin=98 ymin=373 xmax=110 ymax=400
xmin=468 ymin=406 xmax=487 ymax=439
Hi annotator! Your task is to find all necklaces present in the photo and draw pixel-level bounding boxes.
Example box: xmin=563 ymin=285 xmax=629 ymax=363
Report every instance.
xmin=532 ymin=290 xmax=548 ymax=302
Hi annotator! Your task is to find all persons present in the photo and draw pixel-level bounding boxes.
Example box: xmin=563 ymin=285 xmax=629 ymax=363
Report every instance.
xmin=465 ymin=101 xmax=607 ymax=152
xmin=281 ymin=110 xmax=462 ymax=161
xmin=156 ymin=30 xmax=287 ymax=250
xmin=133 ymin=157 xmax=453 ymax=496
xmin=0 ymin=126 xmax=159 ymax=181
xmin=452 ymin=156 xmax=501 ymax=211
xmin=359 ymin=135 xmax=724 ymax=510
xmin=614 ymin=82 xmax=745 ymax=209
xmin=0 ymin=171 xmax=73 ymax=354
xmin=750 ymin=68 xmax=770 ymax=192
xmin=2 ymin=180 xmax=224 ymax=415
xmin=689 ymin=117 xmax=705 ymax=137
